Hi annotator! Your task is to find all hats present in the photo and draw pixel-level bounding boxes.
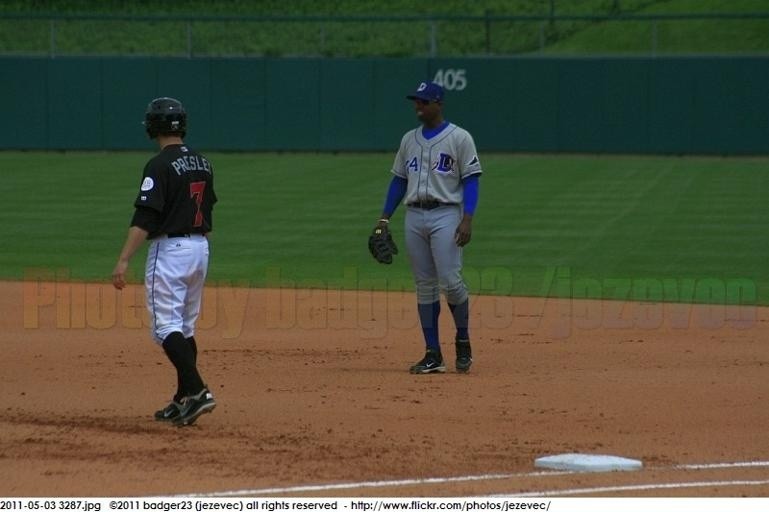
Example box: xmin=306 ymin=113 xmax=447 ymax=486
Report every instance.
xmin=406 ymin=82 xmax=444 ymax=101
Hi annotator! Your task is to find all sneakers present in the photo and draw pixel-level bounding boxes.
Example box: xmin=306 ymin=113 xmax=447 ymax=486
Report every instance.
xmin=410 ymin=345 xmax=446 ymax=373
xmin=154 ymin=387 xmax=216 ymax=426
xmin=456 ymin=337 xmax=473 ymax=374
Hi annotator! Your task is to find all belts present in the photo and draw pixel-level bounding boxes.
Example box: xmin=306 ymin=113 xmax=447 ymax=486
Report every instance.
xmin=408 ymin=201 xmax=456 ymax=209
xmin=167 ymin=232 xmax=205 ymax=238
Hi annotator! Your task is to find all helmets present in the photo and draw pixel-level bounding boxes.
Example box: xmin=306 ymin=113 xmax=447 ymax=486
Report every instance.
xmin=141 ymin=98 xmax=187 ymax=142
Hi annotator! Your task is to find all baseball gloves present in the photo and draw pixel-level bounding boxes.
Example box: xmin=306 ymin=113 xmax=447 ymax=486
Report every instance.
xmin=369 ymin=224 xmax=397 ymax=264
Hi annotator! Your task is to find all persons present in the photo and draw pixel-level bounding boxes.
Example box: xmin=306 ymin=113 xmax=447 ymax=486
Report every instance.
xmin=112 ymin=96 xmax=218 ymax=431
xmin=367 ymin=80 xmax=482 ymax=376
